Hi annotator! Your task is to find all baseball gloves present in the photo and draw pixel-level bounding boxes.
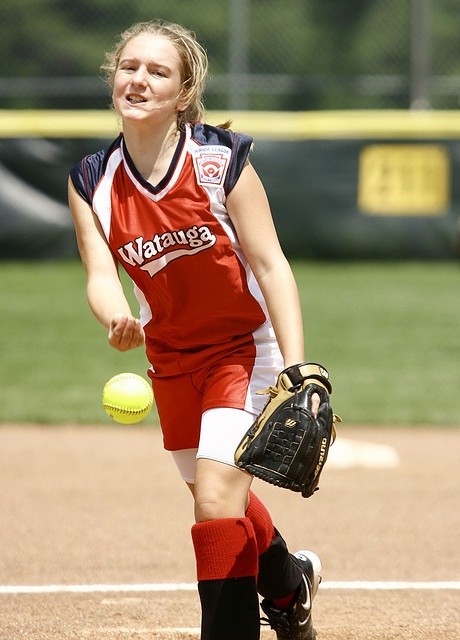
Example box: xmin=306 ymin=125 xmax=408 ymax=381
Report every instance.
xmin=234 ymin=362 xmax=342 ymax=497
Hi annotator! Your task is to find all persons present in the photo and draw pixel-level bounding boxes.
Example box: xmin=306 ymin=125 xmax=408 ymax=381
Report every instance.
xmin=67 ymin=21 xmax=332 ymax=640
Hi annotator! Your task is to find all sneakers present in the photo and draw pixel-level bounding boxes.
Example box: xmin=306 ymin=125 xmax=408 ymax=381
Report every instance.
xmin=258 ymin=550 xmax=322 ymax=639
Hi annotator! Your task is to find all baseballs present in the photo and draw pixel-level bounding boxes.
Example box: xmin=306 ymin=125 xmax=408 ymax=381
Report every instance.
xmin=102 ymin=372 xmax=154 ymax=424
